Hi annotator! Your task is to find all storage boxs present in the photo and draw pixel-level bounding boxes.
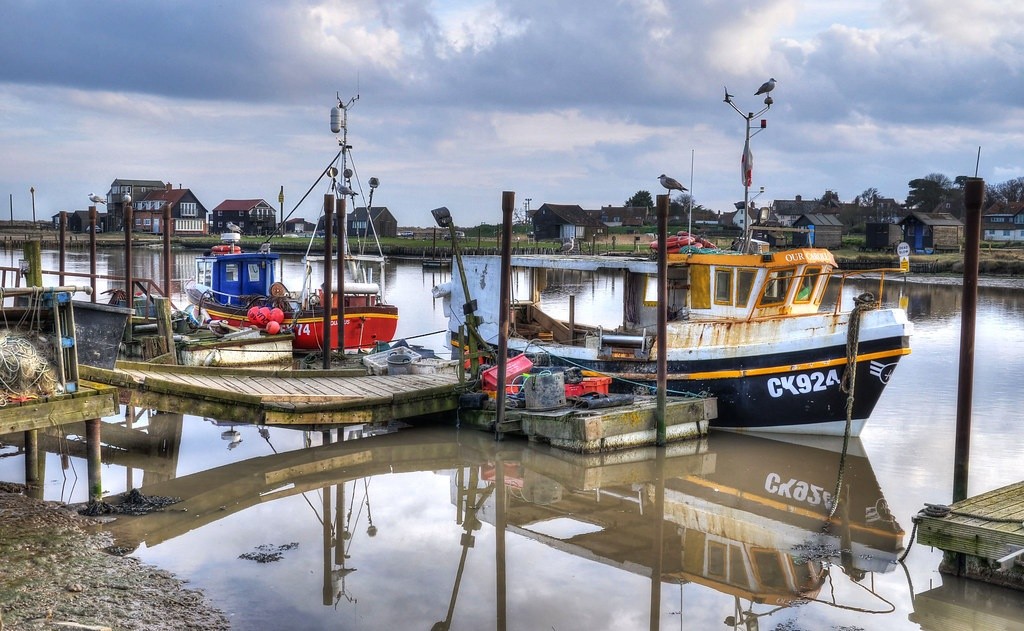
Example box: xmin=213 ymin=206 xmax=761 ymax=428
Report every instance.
xmin=564 ymin=377 xmax=612 ymax=399
xmin=363 ymin=346 xmax=422 ymax=376
xmin=481 ymin=354 xmax=533 ymax=392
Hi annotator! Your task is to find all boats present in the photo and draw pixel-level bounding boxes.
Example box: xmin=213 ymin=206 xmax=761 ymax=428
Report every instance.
xmin=449 ymin=430 xmax=910 ymax=610
xmin=0 ymin=70 xmax=917 ymax=440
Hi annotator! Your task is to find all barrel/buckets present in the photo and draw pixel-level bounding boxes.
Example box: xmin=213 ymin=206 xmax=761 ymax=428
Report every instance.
xmin=386 ymin=352 xmax=412 ymax=376
xmin=523 ymin=371 xmax=566 ymax=411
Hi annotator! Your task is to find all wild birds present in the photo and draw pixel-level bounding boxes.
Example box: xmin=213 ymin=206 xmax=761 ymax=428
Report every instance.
xmin=658 ymin=174 xmax=689 ymax=196
xmin=754 ymin=78 xmax=777 ymax=96
xmin=122 ymin=192 xmax=131 ymax=206
xmin=337 ymin=182 xmax=359 ymax=200
xmin=88 ymin=192 xmax=106 ymax=207
xmin=226 ymin=221 xmax=243 ymax=233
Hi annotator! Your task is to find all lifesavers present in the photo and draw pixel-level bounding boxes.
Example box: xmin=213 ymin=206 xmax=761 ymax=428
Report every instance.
xmin=676 ymin=230 xmax=717 ymax=247
xmin=667 ymin=241 xmax=703 ymax=254
xmin=210 ymin=244 xmax=241 ymax=252
xmin=650 ymin=235 xmax=694 ymax=248
